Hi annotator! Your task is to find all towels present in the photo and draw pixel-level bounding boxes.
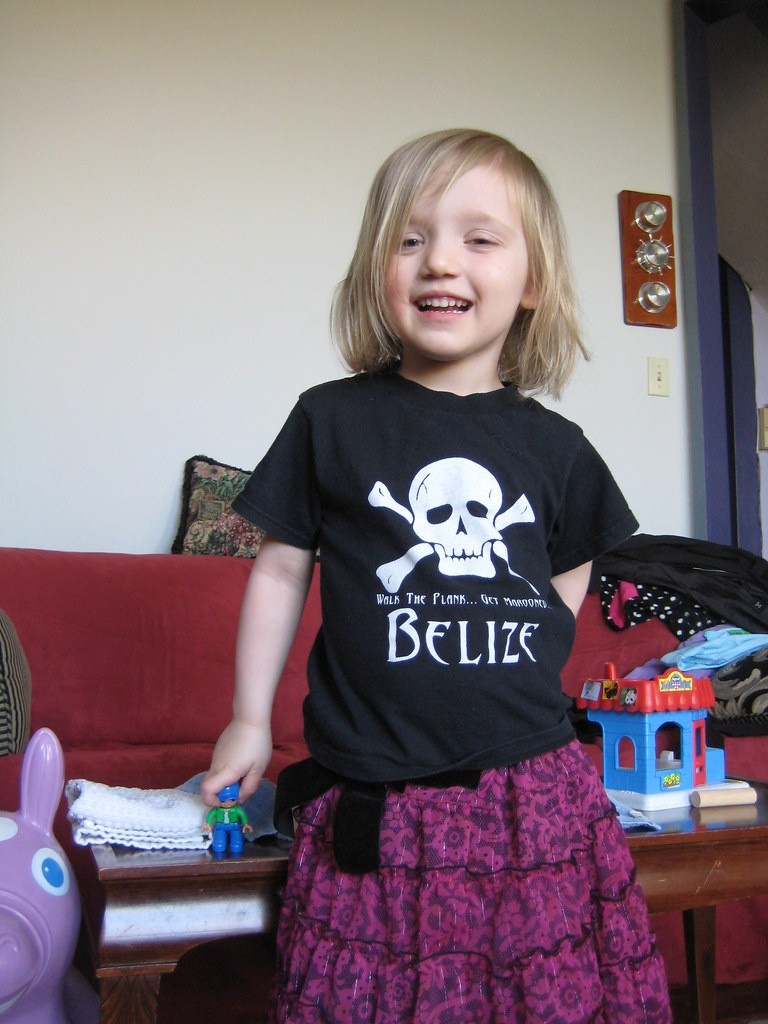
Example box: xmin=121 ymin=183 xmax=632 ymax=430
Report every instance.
xmin=63 ymin=770 xmax=278 ymax=850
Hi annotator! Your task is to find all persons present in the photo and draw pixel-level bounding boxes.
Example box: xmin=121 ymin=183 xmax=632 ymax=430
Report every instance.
xmin=199 ymin=129 xmax=671 ymax=1024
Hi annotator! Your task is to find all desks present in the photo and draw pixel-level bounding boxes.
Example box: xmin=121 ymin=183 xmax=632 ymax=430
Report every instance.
xmin=68 ymin=816 xmax=768 ymax=1024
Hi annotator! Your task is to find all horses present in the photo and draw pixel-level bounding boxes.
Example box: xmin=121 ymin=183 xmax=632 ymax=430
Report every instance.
xmin=1 ymin=727 xmax=99 ymax=1024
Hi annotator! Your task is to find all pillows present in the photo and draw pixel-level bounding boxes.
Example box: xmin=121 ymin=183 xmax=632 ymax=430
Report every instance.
xmin=169 ymin=456 xmax=265 ymax=555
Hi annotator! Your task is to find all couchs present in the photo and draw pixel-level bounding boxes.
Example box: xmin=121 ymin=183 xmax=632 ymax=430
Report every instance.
xmin=0 ymin=545 xmax=767 ymax=983
